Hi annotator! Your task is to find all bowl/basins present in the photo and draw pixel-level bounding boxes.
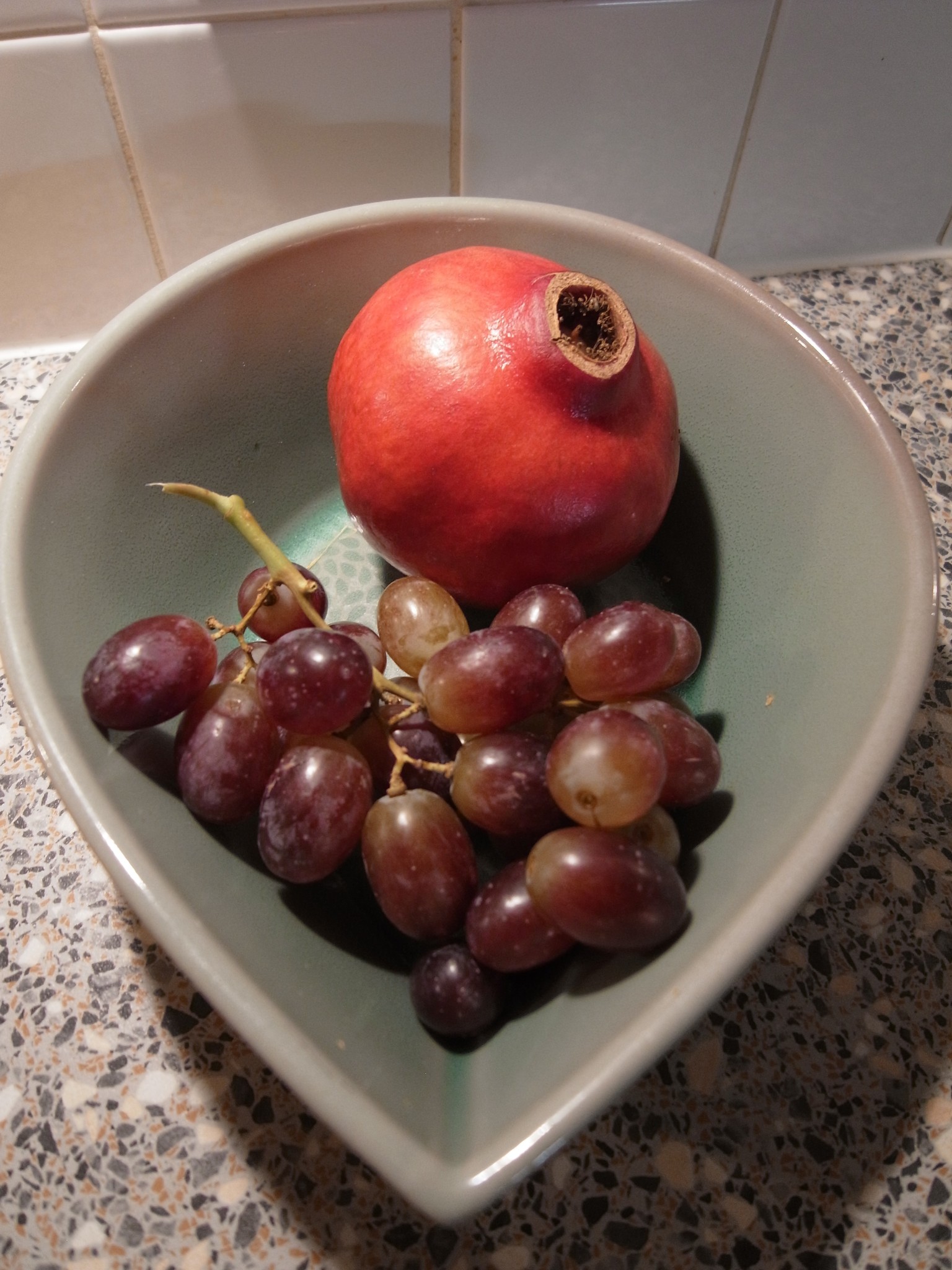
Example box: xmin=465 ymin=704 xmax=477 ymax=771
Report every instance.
xmin=3 ymin=196 xmax=936 ymax=1226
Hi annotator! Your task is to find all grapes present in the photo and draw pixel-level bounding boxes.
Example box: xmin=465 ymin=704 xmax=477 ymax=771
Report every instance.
xmin=79 ymin=562 xmax=724 ymax=1035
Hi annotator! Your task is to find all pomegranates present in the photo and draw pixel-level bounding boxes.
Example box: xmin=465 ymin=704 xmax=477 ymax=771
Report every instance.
xmin=325 ymin=245 xmax=680 ymax=612
